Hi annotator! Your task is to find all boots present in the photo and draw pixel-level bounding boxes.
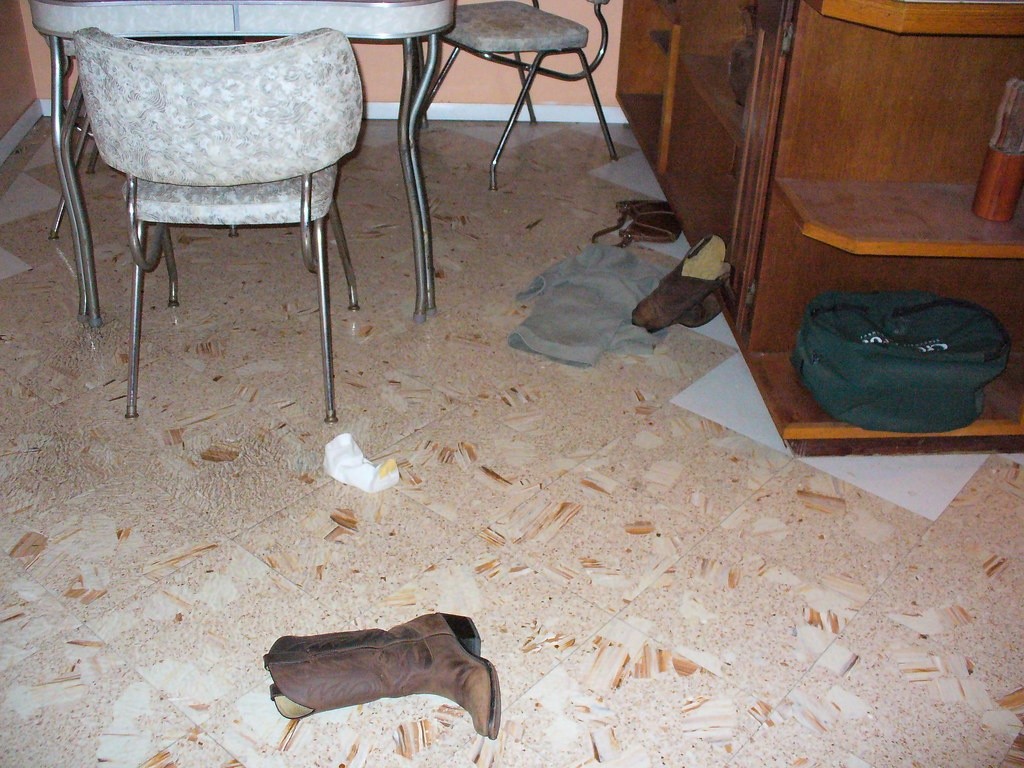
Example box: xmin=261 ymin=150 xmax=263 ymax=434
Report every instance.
xmin=263 ymin=612 xmax=501 ymax=741
xmin=632 ymin=235 xmax=731 ymax=334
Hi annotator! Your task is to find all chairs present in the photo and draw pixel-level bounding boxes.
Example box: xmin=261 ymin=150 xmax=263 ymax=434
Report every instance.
xmin=31 ymin=24 xmax=366 ymax=426
xmin=420 ymin=0 xmax=620 ymax=191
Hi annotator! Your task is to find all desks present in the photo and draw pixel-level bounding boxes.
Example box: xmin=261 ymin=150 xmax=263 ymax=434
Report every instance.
xmin=27 ymin=0 xmax=456 ymax=326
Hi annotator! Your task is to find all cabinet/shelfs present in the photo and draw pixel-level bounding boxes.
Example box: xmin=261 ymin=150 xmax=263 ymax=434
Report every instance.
xmin=614 ymin=0 xmax=1024 ymax=459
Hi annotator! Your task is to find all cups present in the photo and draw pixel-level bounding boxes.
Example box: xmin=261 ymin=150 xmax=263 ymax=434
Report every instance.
xmin=971 ymin=144 xmax=1024 ymax=222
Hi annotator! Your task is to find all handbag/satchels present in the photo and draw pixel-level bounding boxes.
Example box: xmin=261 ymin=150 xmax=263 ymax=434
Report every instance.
xmin=592 ymin=199 xmax=683 ymax=248
xmin=789 ymin=287 xmax=1011 ymax=434
